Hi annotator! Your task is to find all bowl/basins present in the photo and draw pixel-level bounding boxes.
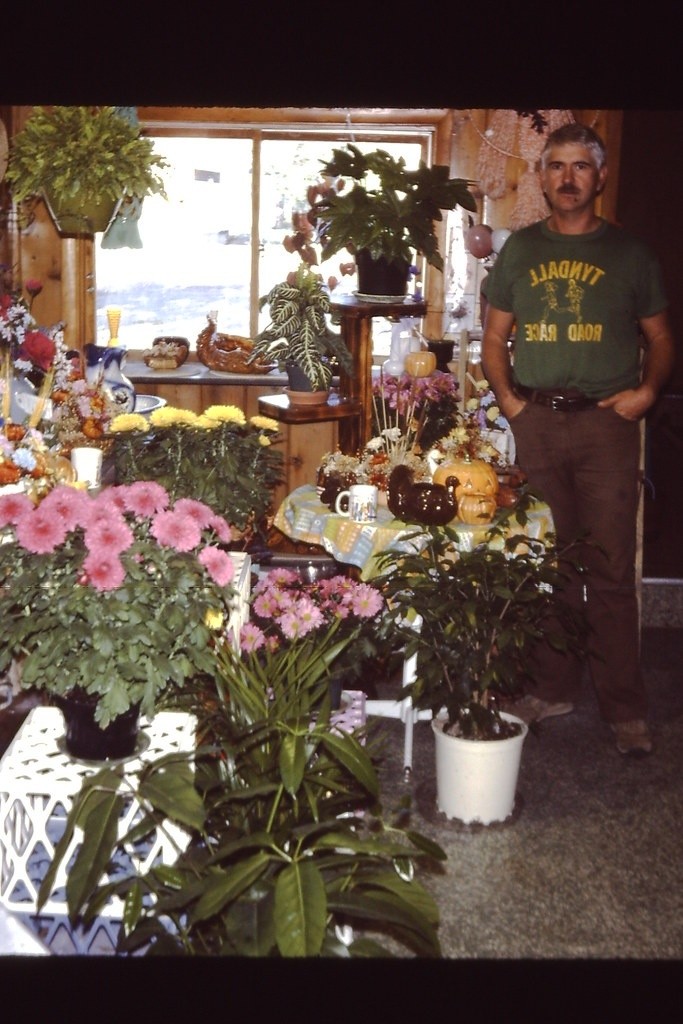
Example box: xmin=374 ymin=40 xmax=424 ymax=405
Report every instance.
xmin=134 ymin=394 xmax=166 ymax=420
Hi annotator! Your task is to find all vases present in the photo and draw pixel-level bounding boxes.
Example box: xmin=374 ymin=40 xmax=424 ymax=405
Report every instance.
xmin=404 ymin=338 xmax=453 ymax=376
xmin=51 ymin=688 xmax=143 ymax=759
xmin=83 ymin=343 xmax=136 ymax=413
xmin=312 ymin=654 xmax=344 ymax=711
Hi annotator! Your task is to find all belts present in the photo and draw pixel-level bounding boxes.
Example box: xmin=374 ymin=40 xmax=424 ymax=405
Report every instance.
xmin=513 ymin=381 xmax=643 ymax=411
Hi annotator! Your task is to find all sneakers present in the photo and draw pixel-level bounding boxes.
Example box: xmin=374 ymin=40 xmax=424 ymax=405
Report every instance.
xmin=610 ymin=722 xmax=656 ymax=757
xmin=506 ymin=696 xmax=576 ymax=725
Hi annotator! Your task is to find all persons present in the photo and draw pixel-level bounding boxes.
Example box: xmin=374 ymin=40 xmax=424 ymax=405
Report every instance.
xmin=480 ymin=124 xmax=675 ymax=755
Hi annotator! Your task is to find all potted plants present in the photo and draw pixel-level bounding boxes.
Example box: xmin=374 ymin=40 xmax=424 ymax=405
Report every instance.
xmin=243 ymin=260 xmax=360 ymax=405
xmin=308 ymin=144 xmax=479 ymax=301
xmin=364 ymin=480 xmax=610 ymax=833
xmin=6 ymin=106 xmax=171 ymax=236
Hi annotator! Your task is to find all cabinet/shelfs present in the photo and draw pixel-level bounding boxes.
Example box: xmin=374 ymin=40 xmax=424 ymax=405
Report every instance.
xmin=258 ymin=292 xmax=425 ymax=458
xmin=0 ymin=706 xmax=199 ymax=954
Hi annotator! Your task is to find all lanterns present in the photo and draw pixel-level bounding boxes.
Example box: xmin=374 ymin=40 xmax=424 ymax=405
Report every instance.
xmin=431 ymin=459 xmax=501 ymax=524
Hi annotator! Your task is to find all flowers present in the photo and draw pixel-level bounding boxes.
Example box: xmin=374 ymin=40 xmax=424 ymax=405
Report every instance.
xmin=319 ymin=366 xmax=508 ymax=492
xmin=1 ymin=269 xmax=290 ymax=726
xmin=439 ymin=302 xmax=468 ymax=340
xmin=242 ymin=564 xmax=384 ymax=654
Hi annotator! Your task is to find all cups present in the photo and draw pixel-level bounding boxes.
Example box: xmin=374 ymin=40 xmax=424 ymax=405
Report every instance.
xmin=334 ymin=486 xmax=377 ymax=522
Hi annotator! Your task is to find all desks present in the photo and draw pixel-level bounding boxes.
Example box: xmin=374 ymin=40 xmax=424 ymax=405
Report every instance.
xmin=272 ymin=484 xmax=560 ymax=782
